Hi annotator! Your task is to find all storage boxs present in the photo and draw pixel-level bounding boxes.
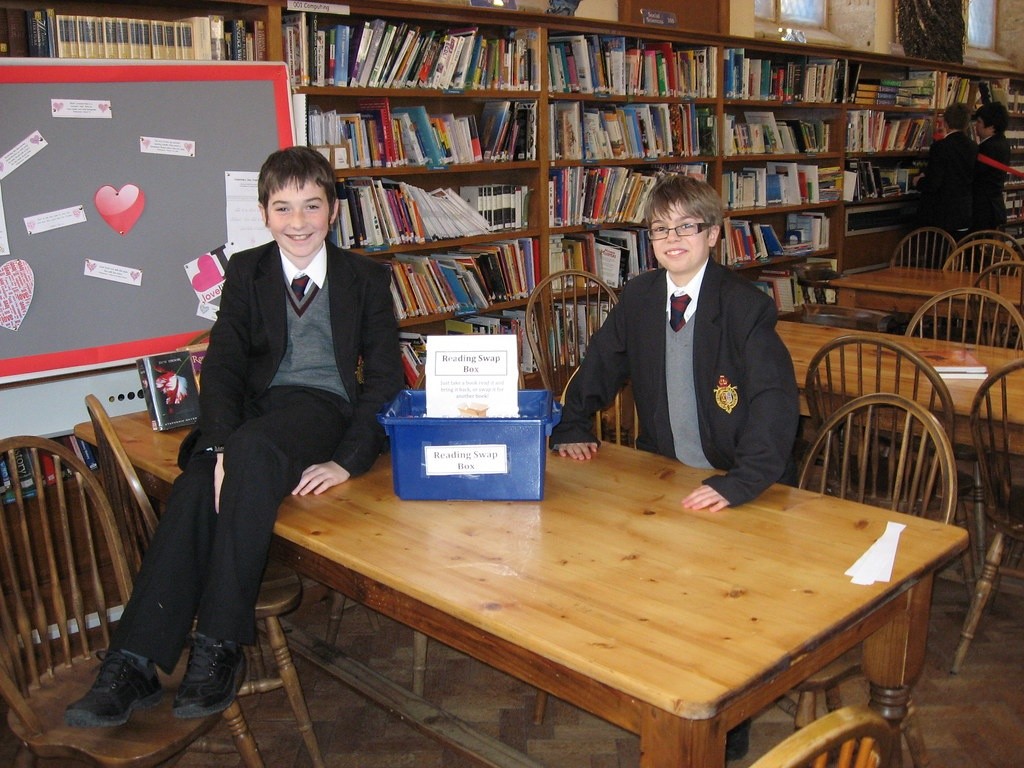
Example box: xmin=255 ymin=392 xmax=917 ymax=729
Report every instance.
xmin=380 ymin=390 xmax=561 ymax=502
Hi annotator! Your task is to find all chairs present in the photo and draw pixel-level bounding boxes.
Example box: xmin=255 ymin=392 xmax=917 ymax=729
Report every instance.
xmin=0 ymin=228 xmax=1023 ymax=768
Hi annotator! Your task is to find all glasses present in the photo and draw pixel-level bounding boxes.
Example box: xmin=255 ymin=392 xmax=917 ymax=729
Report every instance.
xmin=647 ymin=223 xmax=710 ymax=240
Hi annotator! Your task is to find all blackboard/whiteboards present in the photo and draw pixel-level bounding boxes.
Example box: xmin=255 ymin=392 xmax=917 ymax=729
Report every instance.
xmin=0 ymin=57 xmax=297 ymax=387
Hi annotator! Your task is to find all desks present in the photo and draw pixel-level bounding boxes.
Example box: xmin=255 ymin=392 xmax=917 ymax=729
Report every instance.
xmin=74 ymin=408 xmax=970 ymax=768
xmin=828 ymin=265 xmax=1023 ymax=338
xmin=770 ymin=322 xmax=1022 ymax=466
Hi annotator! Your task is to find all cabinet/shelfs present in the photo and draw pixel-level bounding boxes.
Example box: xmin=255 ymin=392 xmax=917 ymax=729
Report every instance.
xmin=719 ymin=31 xmax=852 ymax=274
xmin=277 ymin=0 xmax=551 ymax=407
xmin=943 ymin=64 xmax=1024 ymax=224
xmin=1 ymin=1 xmax=280 ymax=59
xmin=850 ymin=49 xmax=941 ymax=228
xmin=542 ymin=17 xmax=726 ymax=373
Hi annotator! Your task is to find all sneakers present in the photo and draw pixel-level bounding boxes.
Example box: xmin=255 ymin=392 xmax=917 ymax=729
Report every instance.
xmin=171 ymin=633 xmax=247 ymax=720
xmin=64 ymin=649 xmax=164 ymax=728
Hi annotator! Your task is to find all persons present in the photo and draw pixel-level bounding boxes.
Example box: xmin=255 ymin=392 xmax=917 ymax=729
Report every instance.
xmin=64 ymin=146 xmax=405 ymax=727
xmin=975 ymin=101 xmax=1011 ymax=273
xmin=912 ymin=102 xmax=980 ymax=270
xmin=548 ymin=174 xmax=799 ymax=513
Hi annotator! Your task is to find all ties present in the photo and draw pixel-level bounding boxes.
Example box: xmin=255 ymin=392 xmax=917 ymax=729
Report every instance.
xmin=670 ymin=294 xmax=691 ymax=332
xmin=291 ymin=276 xmax=309 ymax=302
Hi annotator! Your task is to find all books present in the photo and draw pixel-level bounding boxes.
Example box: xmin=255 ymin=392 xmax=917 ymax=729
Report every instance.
xmin=548 ymin=228 xmax=665 ymax=289
xmin=330 ymin=176 xmax=534 ymax=250
xmin=548 ymin=300 xmax=613 ymax=371
xmin=293 ymin=94 xmax=537 ymax=168
xmin=721 ymin=46 xmax=849 ymax=313
xmin=549 ymin=35 xmax=718 ymax=98
xmin=282 ymin=12 xmax=542 ymax=93
xmin=367 ymin=237 xmax=541 ymax=320
xmin=0 ymin=8 xmax=267 ymax=62
xmin=135 ymin=343 xmax=209 ymax=433
xmin=843 ymin=60 xmax=1024 ymax=258
xmin=0 ymin=434 xmax=98 ymax=505
xmin=548 ymin=162 xmax=708 ymax=228
xmin=549 ymin=100 xmax=719 ymax=162
xmin=918 ymin=349 xmax=990 ymax=380
xmin=397 ymin=310 xmax=541 ymax=391
